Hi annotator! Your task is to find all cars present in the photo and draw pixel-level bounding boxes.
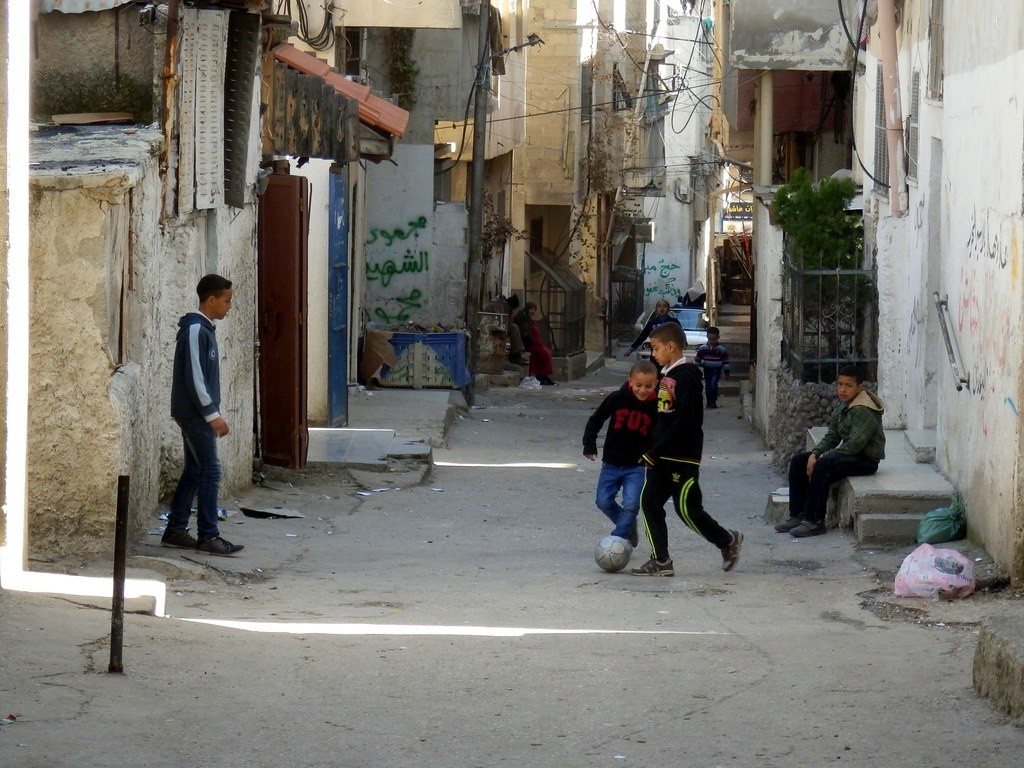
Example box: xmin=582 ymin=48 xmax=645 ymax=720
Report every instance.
xmin=642 ymin=308 xmax=709 ymax=352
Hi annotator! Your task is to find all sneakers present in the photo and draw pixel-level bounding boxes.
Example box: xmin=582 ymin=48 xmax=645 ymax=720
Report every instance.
xmin=161 ymin=524 xmax=198 ymax=550
xmin=632 ymin=559 xmax=675 ymax=577
xmin=196 ymin=534 xmax=244 ymax=556
xmin=789 ymin=518 xmax=826 ymax=537
xmin=775 ymin=513 xmax=803 ymax=532
xmin=721 ymin=529 xmax=743 ymax=572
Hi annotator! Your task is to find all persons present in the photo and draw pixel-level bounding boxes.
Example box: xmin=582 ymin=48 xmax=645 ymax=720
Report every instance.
xmin=774 ymin=366 xmax=885 ymax=537
xmin=514 ymin=302 xmax=559 ymax=386
xmin=583 ymin=361 xmax=660 ymax=547
xmin=695 ymin=326 xmax=730 ymax=409
xmin=159 ymin=274 xmax=244 ymax=556
xmin=632 ymin=323 xmax=744 ymax=577
xmin=484 ymin=296 xmax=529 ymax=367
xmin=624 ymin=300 xmax=688 ymax=381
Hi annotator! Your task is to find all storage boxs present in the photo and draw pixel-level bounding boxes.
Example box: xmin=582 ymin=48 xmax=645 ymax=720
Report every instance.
xmin=363 ymin=321 xmax=472 ymax=388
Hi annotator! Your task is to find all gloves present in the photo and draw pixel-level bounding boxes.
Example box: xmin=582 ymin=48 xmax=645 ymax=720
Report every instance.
xmin=624 ymin=347 xmax=635 ymax=358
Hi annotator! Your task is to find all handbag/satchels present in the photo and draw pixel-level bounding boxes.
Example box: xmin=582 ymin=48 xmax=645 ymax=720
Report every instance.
xmin=894 ymin=542 xmax=975 ymax=598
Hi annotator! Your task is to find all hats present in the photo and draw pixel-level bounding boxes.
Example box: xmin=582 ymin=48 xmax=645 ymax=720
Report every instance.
xmin=507 ymin=293 xmax=519 ymax=308
xmin=526 ymin=301 xmax=538 ymax=310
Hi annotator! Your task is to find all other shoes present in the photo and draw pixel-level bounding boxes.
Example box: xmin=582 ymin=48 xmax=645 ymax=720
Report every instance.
xmin=538 ymin=376 xmax=555 ymax=385
xmin=705 ymin=399 xmax=718 ymax=410
xmin=509 ymin=355 xmax=529 ymax=366
xmin=628 ymin=518 xmax=639 ymax=548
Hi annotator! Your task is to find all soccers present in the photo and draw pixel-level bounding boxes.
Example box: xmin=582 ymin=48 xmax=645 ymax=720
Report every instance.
xmin=595 ymin=535 xmax=632 ymax=573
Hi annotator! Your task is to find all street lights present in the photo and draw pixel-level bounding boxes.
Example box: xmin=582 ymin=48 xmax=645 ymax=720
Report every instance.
xmin=460 ymin=30 xmax=546 ymax=405
